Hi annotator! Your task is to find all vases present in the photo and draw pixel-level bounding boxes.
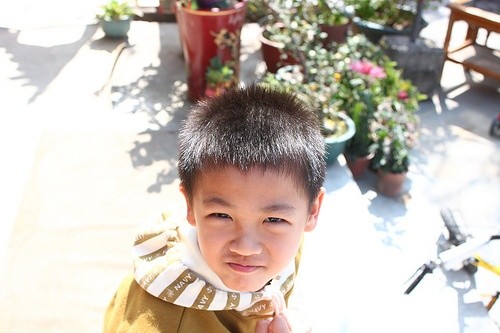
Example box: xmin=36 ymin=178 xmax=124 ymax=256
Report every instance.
xmin=345 ymin=148 xmax=371 ymax=178
xmin=374 ymin=166 xmax=409 ymax=198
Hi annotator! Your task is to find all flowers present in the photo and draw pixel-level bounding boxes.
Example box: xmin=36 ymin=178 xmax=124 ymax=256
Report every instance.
xmin=369 ymin=91 xmax=419 ymax=170
xmin=345 ymin=61 xmax=388 ymax=153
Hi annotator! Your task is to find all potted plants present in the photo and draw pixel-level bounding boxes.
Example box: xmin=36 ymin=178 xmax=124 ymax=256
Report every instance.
xmin=177 ymin=0 xmax=246 ymax=100
xmin=261 ymin=0 xmax=352 ymax=161
xmin=355 ymin=0 xmax=429 ymax=47
xmin=99 ymin=2 xmax=132 ymax=38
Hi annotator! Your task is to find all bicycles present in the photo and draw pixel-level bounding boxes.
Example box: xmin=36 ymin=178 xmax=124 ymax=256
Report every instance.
xmin=403 ymin=205 xmax=500 ymax=311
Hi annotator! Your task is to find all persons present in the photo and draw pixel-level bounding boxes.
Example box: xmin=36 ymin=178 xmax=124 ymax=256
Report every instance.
xmin=104 ymin=80 xmax=327 ymax=333
